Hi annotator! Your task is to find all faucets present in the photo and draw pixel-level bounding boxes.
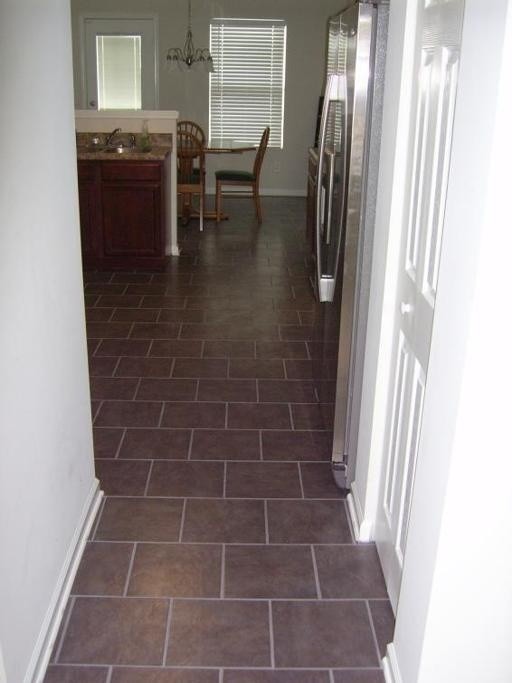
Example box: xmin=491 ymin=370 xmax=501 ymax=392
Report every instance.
xmin=106 ymin=128 xmax=121 ymax=145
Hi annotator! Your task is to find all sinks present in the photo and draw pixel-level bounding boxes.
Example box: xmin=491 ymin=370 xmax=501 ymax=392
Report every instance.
xmin=77 ymin=146 xmax=103 ymax=154
xmin=104 ymin=147 xmax=151 ymax=154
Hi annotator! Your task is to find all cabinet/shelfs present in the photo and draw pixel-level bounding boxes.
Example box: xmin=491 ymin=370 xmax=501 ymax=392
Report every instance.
xmin=77 ymin=161 xmax=162 ymax=259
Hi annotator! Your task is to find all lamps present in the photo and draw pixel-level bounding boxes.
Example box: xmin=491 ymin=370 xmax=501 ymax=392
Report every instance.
xmin=167 ymin=0 xmax=214 ymax=68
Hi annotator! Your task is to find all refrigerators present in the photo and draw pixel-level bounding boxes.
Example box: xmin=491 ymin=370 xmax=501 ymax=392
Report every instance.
xmin=317 ymin=1 xmax=376 ymax=489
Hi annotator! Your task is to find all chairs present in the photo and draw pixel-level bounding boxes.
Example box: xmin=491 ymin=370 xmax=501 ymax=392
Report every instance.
xmin=177 ymin=119 xmax=270 ymax=231
